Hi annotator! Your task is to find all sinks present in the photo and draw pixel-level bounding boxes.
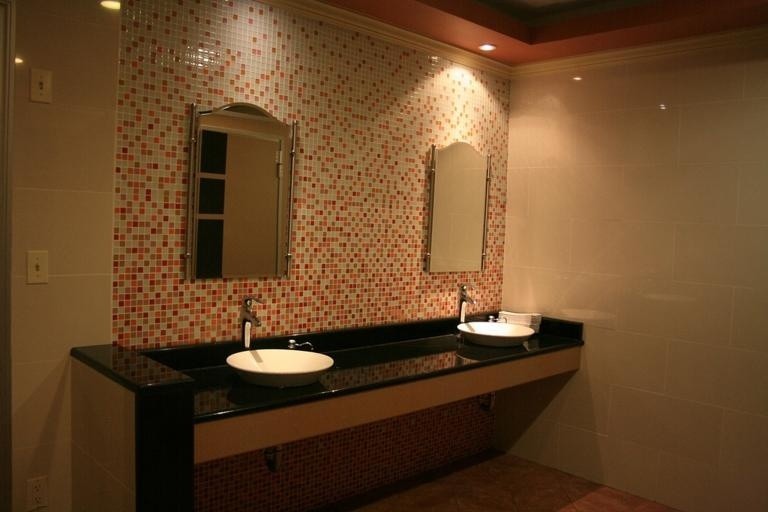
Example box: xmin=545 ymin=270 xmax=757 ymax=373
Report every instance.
xmin=455 ymin=321 xmax=536 ymax=349
xmin=225 ymin=348 xmax=336 ymax=389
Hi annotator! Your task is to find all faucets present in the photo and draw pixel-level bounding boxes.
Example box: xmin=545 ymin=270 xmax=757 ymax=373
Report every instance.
xmin=458 ymin=284 xmax=477 ymax=323
xmin=239 ymin=296 xmax=266 ymax=350
xmin=288 ymin=339 xmax=314 ymax=351
xmin=487 ymin=315 xmax=508 ymax=323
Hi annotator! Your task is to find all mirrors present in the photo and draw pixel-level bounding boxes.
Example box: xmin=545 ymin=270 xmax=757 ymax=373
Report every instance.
xmin=185 ymin=101 xmax=298 ymax=283
xmin=425 ymin=140 xmax=491 ymax=275
